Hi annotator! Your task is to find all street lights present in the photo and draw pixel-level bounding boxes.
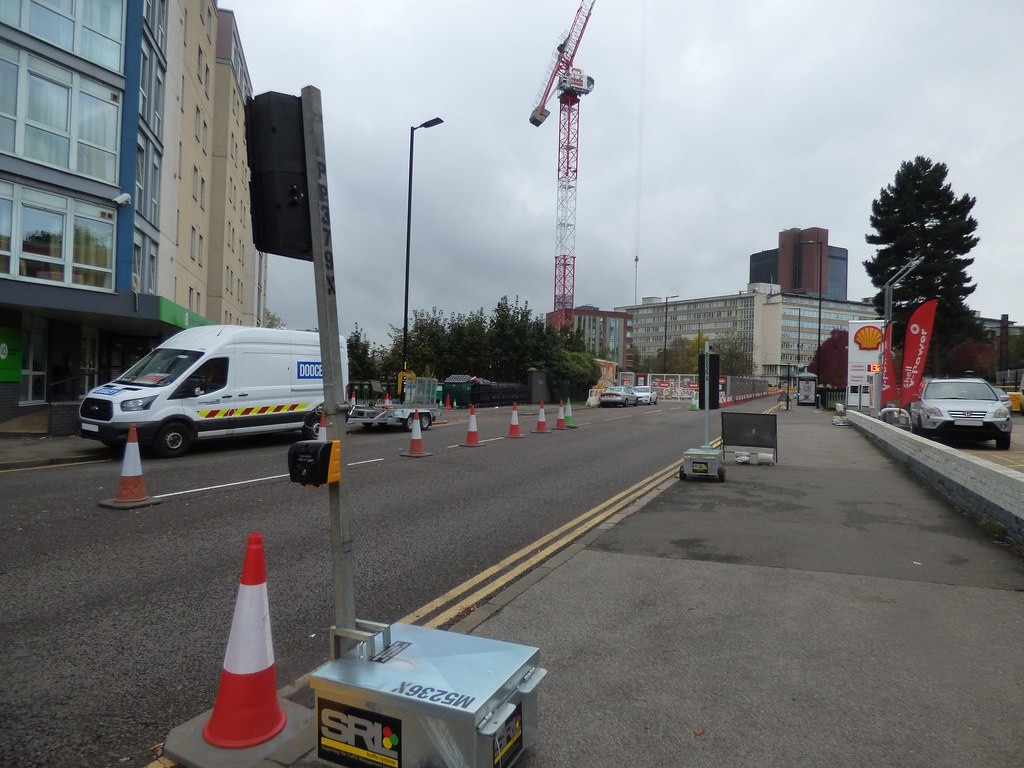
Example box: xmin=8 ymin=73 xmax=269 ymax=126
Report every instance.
xmin=401 ymin=117 xmax=446 ymax=403
xmin=664 ymin=295 xmax=679 ymax=373
xmin=797 ymin=240 xmax=823 ymax=409
xmin=634 ymin=254 xmax=639 ymax=306
xmin=787 ymin=307 xmax=801 ymax=376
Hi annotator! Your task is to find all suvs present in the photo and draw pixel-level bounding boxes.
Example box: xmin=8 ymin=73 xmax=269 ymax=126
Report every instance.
xmin=909 ymin=376 xmax=1013 ymax=452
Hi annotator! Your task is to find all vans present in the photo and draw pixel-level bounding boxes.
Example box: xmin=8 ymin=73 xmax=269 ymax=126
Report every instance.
xmin=77 ymin=324 xmax=351 ymax=458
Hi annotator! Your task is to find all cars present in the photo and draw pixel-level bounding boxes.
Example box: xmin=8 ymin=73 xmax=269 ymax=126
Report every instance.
xmin=634 ymin=386 xmax=658 ymax=406
xmin=599 ymin=386 xmax=639 ymax=408
xmin=994 ymin=388 xmax=1012 ymax=415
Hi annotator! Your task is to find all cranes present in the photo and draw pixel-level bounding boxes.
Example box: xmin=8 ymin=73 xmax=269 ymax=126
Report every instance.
xmin=528 ymin=0 xmax=600 ymax=340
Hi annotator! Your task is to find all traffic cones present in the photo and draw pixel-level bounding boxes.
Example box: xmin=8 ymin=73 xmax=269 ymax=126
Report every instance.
xmin=459 ymin=405 xmax=486 ymax=448
xmin=501 ymin=402 xmax=527 ymax=439
xmin=400 ymin=410 xmax=434 ymax=458
xmin=550 ymin=400 xmax=569 ymax=431
xmin=443 ymin=395 xmax=452 ymax=412
xmin=96 ymin=423 xmax=163 ymax=509
xmin=384 ymin=393 xmax=391 ymax=408
xmin=531 ymin=401 xmax=552 ymax=434
xmin=202 ymin=532 xmax=287 ymax=749
xmin=565 ymin=398 xmax=578 ymax=429
xmin=350 ymin=391 xmax=357 ymax=408
xmin=318 ymin=413 xmax=328 ymax=446
